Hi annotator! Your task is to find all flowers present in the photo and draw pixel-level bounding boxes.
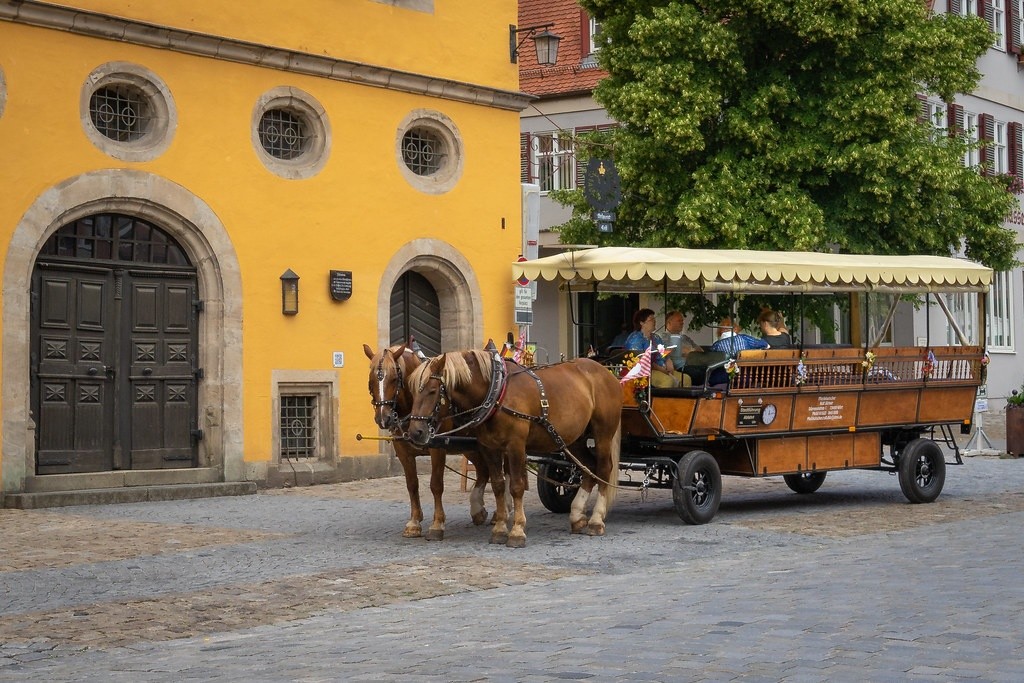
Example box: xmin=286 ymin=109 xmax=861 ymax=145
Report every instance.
xmin=861 ymin=351 xmax=877 ymax=383
xmin=981 ymin=357 xmax=991 ymax=385
xmin=725 ymin=359 xmax=739 ymax=380
xmin=921 ymin=351 xmax=938 ymax=379
xmin=795 ymin=352 xmax=807 ymax=385
xmin=606 ymin=352 xmax=648 ymax=404
xmin=1005 ymin=170 xmax=1024 ymax=195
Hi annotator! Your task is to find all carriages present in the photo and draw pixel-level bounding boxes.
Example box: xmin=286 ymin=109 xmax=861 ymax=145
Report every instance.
xmin=362 ymin=245 xmax=994 ymax=545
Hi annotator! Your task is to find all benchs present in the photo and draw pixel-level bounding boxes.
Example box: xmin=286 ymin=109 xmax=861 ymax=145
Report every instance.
xmin=606 ymin=344 xmax=852 ymax=364
xmin=730 ymin=346 xmax=982 ymax=393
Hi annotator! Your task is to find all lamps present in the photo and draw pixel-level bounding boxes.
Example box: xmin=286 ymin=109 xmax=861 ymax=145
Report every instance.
xmin=280 ymin=269 xmax=300 ymax=315
xmin=510 ymin=22 xmax=560 ymax=67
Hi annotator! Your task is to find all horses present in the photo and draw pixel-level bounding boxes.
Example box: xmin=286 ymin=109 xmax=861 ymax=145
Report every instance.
xmin=362 ymin=340 xmax=626 ymax=549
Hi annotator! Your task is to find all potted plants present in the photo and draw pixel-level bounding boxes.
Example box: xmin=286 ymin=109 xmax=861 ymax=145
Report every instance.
xmin=1018 ymin=43 xmax=1024 ymax=61
xmin=1002 ymin=384 xmax=1024 ymax=459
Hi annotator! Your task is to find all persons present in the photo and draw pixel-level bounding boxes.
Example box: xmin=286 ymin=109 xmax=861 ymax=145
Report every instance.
xmin=660 ymin=312 xmax=707 ymax=386
xmin=707 ymin=318 xmax=770 ymax=387
xmin=758 ymin=310 xmax=792 ymax=386
xmin=626 ymin=307 xmax=692 ymax=388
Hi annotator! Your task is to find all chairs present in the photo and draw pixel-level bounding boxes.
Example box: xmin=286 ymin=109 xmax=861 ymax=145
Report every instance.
xmin=652 ymin=351 xmax=730 ymax=399
xmin=613 ymin=350 xmax=644 ymax=366
